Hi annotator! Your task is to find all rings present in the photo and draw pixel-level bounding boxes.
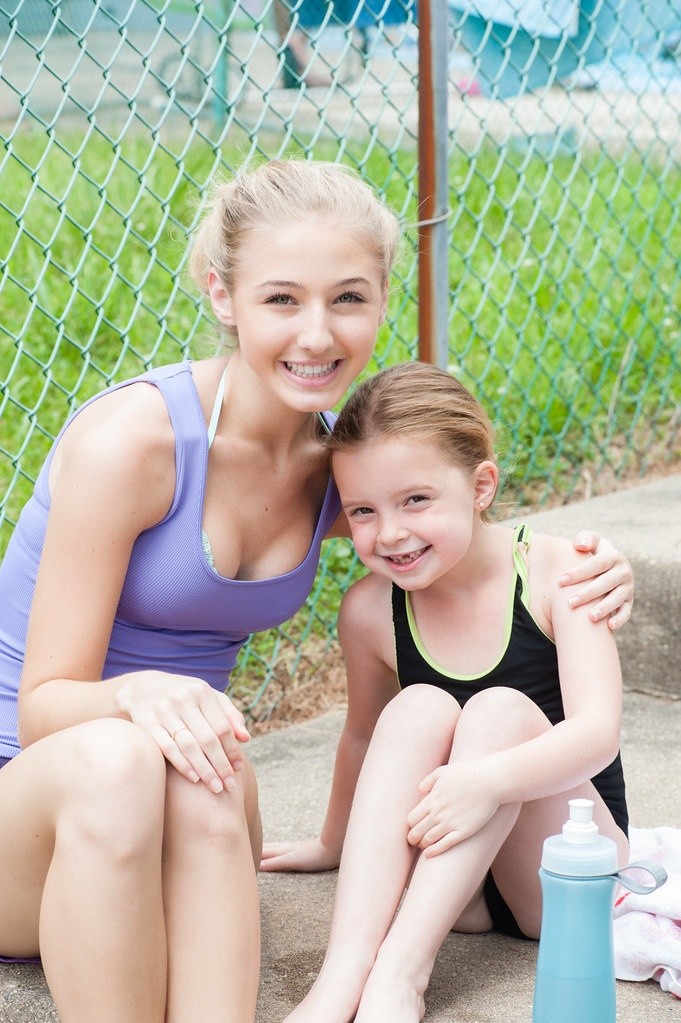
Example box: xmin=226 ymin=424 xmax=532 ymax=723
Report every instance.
xmin=172 ymin=727 xmax=190 ymax=740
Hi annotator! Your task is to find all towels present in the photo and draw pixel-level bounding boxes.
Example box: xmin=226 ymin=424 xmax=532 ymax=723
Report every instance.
xmin=613 ymin=825 xmax=681 ymax=998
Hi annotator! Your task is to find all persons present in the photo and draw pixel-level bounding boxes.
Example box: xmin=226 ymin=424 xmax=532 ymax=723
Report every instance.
xmin=0 ymin=157 xmax=635 ymax=1023
xmin=259 ymin=361 xmax=631 ymax=1023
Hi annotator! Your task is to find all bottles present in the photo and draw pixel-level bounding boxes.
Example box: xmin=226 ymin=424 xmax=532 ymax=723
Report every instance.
xmin=532 ymin=797 xmax=668 ymax=1023
